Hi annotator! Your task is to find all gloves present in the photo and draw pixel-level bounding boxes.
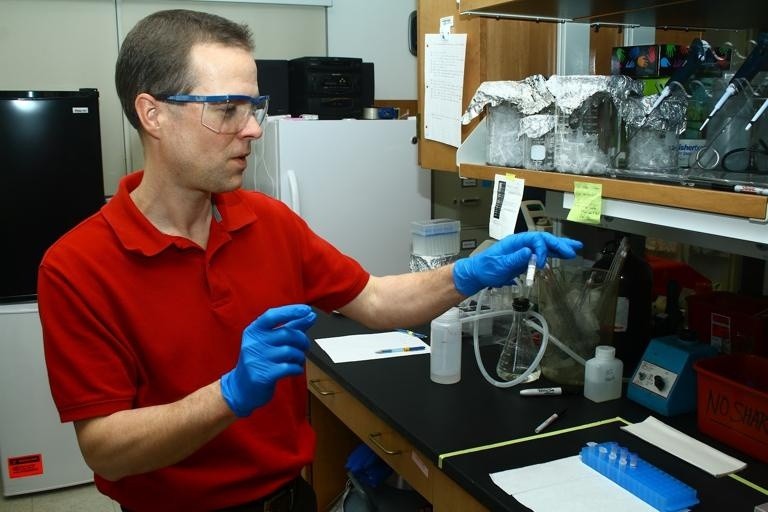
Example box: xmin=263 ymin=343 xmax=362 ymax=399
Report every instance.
xmin=453 ymin=230 xmax=584 ymax=298
xmin=220 ymin=303 xmax=316 ymax=418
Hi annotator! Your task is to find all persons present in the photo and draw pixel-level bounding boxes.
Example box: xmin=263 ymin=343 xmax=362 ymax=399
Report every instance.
xmin=34 ymin=11 xmax=584 ymax=509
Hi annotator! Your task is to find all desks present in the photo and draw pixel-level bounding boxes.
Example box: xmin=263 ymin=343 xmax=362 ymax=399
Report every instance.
xmin=302 ymin=305 xmax=767 ymax=510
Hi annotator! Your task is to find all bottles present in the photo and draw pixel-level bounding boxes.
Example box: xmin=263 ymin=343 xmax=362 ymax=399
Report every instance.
xmin=584 ymin=442 xmax=638 ymax=484
xmin=430 ymin=312 xmax=463 ymax=385
xmin=582 ymin=345 xmax=625 ymax=404
xmin=495 ymin=300 xmax=544 ymax=386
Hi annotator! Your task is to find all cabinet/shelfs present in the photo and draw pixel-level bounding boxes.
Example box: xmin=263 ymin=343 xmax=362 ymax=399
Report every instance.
xmin=417 ymin=0 xmax=558 ymax=173
xmin=454 ymin=0 xmax=767 ymax=219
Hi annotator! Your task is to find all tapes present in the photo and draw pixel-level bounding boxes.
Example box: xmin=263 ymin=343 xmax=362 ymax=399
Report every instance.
xmin=362 ymin=107 xmax=396 ymax=119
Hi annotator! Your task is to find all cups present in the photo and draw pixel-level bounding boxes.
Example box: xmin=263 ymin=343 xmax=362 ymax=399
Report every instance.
xmin=536 ymin=265 xmax=623 ymax=386
xmin=474 ymin=78 xmax=680 ymax=180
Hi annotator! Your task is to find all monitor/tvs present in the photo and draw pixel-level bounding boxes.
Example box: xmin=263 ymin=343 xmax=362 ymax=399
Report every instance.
xmin=0 ymin=90 xmax=106 ymax=303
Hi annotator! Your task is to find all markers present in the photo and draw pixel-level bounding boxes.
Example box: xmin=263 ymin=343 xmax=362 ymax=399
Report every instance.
xmin=519 ymin=387 xmax=581 ymax=396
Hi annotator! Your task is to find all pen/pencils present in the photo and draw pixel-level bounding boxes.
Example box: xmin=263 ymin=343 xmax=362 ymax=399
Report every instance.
xmin=399 ymin=329 xmax=428 ymax=339
xmin=534 ymin=408 xmax=568 ymax=434
xmin=376 ymin=346 xmax=425 ymax=353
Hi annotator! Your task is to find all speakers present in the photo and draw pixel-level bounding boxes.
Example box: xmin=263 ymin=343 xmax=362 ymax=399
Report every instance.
xmin=362 ymin=63 xmax=374 ymax=107
xmin=256 ymin=59 xmax=289 ymax=116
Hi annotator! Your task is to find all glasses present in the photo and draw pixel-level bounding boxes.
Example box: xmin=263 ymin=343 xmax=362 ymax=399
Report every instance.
xmin=168 ymin=94 xmax=271 ymax=137
